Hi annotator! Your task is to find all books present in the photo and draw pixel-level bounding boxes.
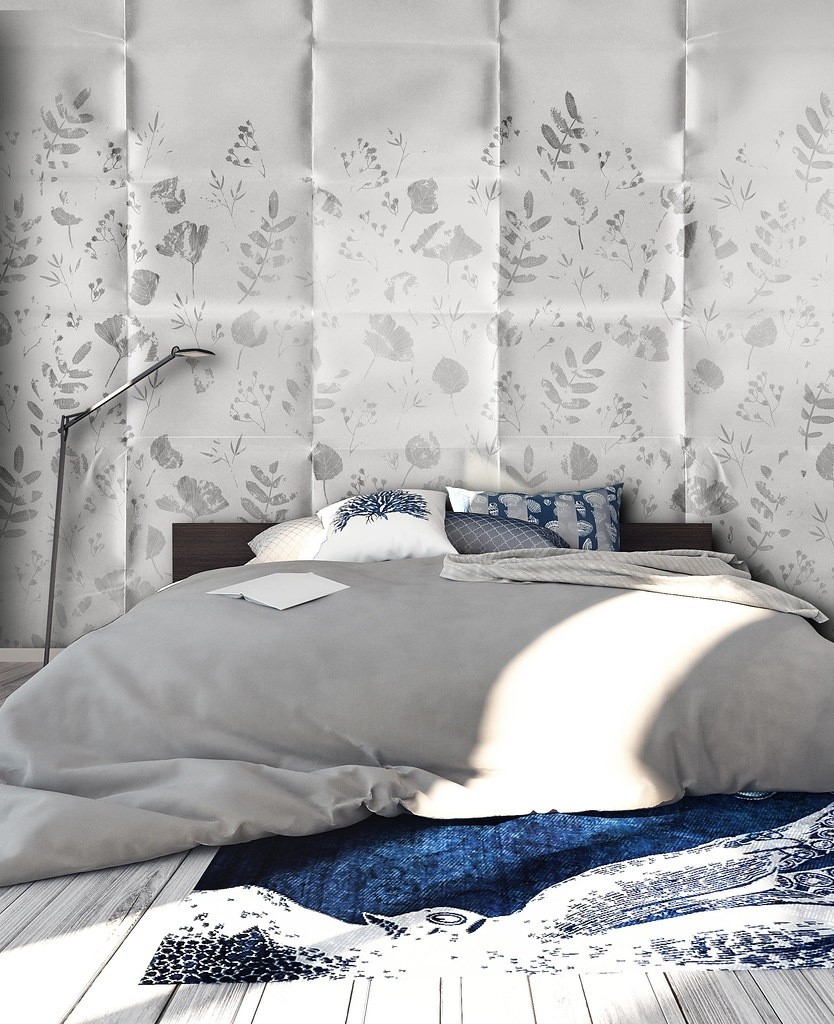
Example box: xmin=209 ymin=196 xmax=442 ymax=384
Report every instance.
xmin=207 ymin=572 xmax=350 ymax=610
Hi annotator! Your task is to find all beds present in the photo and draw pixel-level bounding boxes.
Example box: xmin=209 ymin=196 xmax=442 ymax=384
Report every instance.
xmin=0 ymin=522 xmax=834 ymax=894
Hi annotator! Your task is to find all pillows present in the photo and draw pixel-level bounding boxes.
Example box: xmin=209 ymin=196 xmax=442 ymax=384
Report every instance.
xmin=444 ymin=482 xmax=625 ymax=551
xmin=246 ymin=515 xmax=326 ymax=563
xmin=315 ymin=489 xmax=460 ymax=560
xmin=444 ymin=512 xmax=571 ymax=555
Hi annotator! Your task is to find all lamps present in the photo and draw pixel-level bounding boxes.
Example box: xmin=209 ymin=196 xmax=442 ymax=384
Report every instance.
xmin=41 ymin=345 xmax=215 ymax=665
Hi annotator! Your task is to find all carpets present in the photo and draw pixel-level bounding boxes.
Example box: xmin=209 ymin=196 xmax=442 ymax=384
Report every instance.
xmin=139 ymin=788 xmax=834 ymax=990
